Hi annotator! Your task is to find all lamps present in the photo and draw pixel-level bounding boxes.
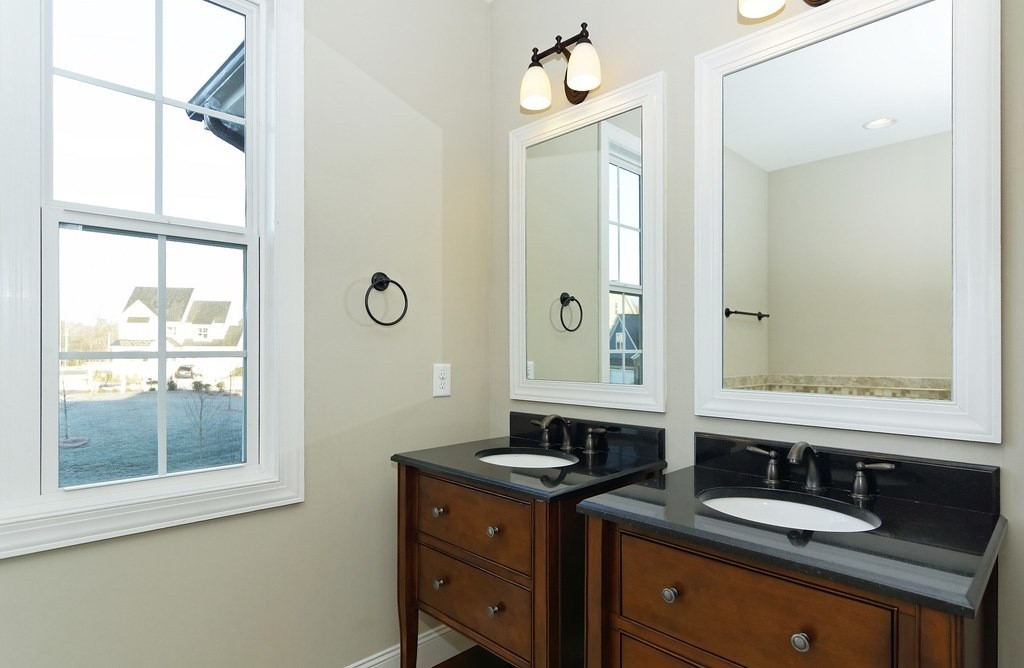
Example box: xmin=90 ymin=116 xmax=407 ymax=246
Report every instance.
xmin=738 ymin=0 xmax=830 ymax=19
xmin=519 ymin=22 xmax=602 ymax=111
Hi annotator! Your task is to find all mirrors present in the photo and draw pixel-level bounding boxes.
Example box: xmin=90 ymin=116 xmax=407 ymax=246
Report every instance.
xmin=508 ymin=70 xmax=668 ymax=413
xmin=692 ymin=0 xmax=1003 ymax=444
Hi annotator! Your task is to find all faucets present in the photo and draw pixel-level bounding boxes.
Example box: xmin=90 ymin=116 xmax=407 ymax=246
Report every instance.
xmin=785 ymin=442 xmax=828 ymax=495
xmin=538 ymin=413 xmax=578 ymax=453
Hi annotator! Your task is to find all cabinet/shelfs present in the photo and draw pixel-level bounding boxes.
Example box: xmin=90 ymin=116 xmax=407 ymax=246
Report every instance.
xmin=396 ymin=462 xmax=586 ymax=668
xmin=584 ymin=515 xmax=998 ymax=668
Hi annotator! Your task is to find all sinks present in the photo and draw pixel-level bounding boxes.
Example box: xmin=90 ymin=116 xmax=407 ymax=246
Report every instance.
xmin=697 ymin=485 xmax=884 ymax=536
xmin=474 ymin=447 xmax=581 ymax=469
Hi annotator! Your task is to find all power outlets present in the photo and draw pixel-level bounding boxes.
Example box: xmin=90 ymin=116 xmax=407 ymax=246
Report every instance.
xmin=432 ymin=364 xmax=451 ymax=397
xmin=527 ymin=362 xmax=534 ymax=380
